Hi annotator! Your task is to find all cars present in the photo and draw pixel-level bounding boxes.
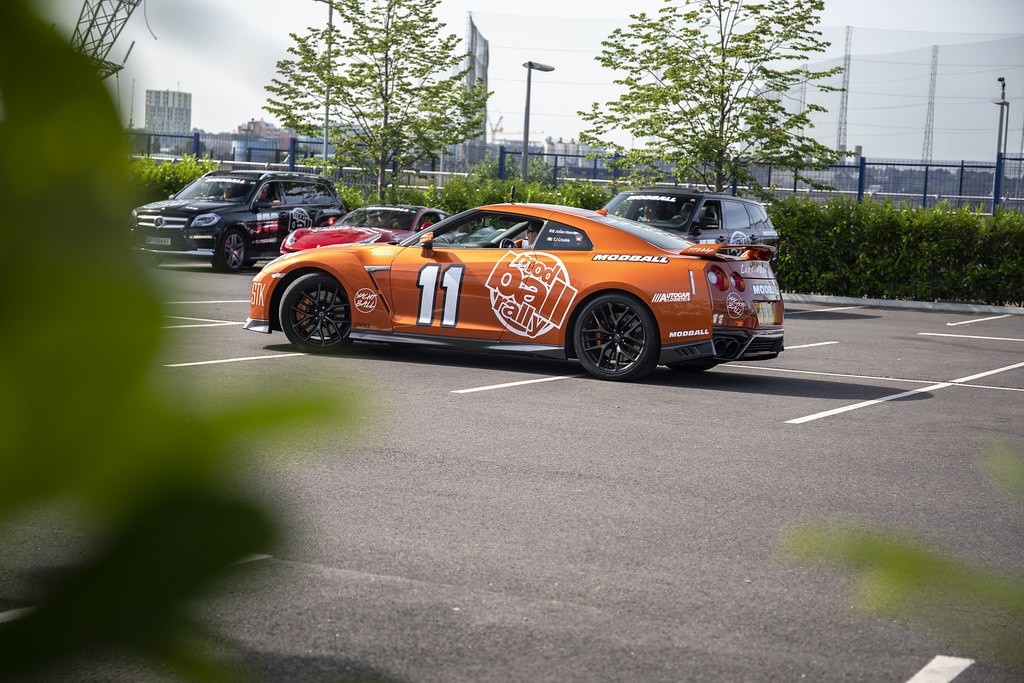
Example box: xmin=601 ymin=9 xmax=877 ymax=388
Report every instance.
xmin=278 ymin=204 xmax=452 ymax=256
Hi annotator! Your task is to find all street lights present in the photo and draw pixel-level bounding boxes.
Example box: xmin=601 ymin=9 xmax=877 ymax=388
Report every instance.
xmin=521 ymin=59 xmax=556 ymax=184
xmin=990 ymin=97 xmax=1010 ymax=218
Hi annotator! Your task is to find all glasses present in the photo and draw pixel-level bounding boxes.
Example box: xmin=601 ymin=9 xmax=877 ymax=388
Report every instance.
xmin=527 ymin=230 xmax=536 ymax=234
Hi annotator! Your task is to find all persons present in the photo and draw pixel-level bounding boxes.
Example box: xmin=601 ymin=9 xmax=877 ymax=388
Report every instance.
xmin=671 ymin=203 xmax=699 ymax=222
xmin=257 ymin=184 xmax=280 ymax=204
xmin=508 ymin=219 xmax=544 ymax=249
xmin=215 ymin=188 xmax=235 ymax=200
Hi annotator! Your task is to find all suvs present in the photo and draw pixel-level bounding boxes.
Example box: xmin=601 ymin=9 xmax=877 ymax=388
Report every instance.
xmin=593 ymin=186 xmax=781 ymax=282
xmin=130 ymin=168 xmax=350 ymax=274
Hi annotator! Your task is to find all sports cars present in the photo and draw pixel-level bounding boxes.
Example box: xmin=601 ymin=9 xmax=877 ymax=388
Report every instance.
xmin=242 ymin=203 xmax=761 ymax=382
